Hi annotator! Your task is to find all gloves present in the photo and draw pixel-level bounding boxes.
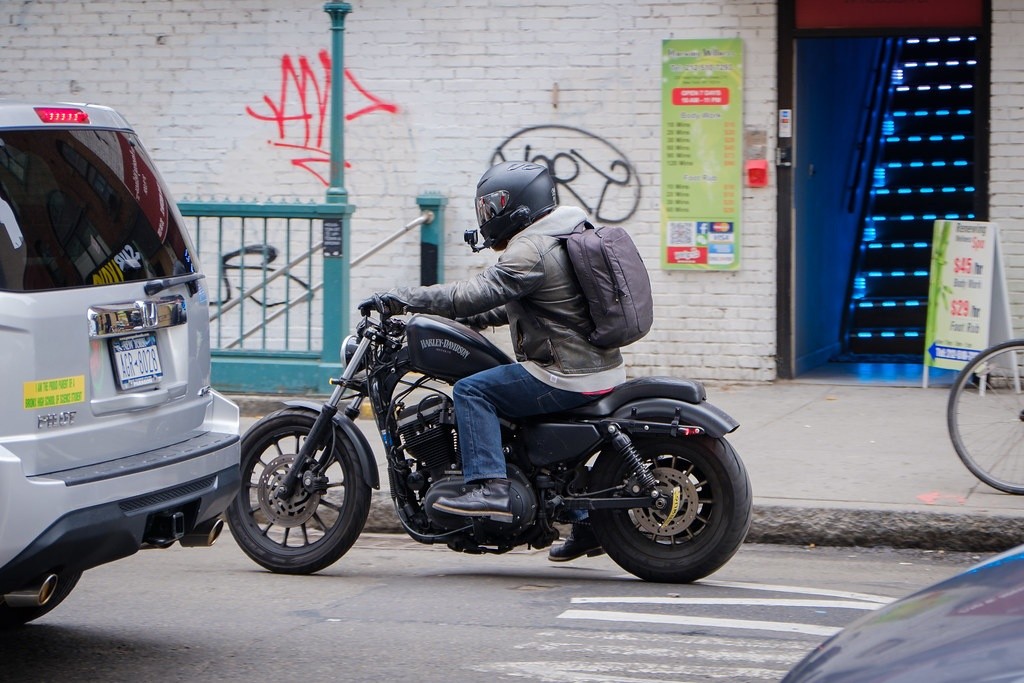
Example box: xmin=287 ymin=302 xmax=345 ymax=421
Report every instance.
xmin=358 ymin=292 xmax=396 ymax=317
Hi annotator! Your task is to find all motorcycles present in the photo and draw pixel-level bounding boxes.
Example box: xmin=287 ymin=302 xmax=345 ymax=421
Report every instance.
xmin=226 ymin=298 xmax=756 ymax=585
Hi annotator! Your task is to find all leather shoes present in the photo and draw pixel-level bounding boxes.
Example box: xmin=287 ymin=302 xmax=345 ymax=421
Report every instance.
xmin=548 ymin=520 xmax=607 ymax=562
xmin=432 ymin=478 xmax=513 ymax=524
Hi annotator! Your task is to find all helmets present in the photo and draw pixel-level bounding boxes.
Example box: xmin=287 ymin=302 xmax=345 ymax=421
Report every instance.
xmin=475 ymin=161 xmax=556 ymax=251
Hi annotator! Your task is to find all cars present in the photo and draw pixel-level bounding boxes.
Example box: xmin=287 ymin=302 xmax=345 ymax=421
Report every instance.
xmin=775 ymin=542 xmax=1024 ymax=683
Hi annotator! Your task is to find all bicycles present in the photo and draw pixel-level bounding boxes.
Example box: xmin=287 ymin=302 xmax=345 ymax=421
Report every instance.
xmin=943 ymin=338 xmax=1023 ymax=499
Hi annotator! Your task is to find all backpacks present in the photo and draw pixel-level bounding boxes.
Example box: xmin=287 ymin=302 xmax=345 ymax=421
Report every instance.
xmin=521 ymin=220 xmax=653 ymax=350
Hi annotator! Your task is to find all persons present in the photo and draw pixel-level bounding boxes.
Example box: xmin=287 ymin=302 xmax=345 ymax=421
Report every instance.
xmin=358 ymin=160 xmax=626 ymax=562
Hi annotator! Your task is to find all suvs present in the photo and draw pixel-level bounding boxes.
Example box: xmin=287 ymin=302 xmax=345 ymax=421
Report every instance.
xmin=0 ymin=104 xmax=253 ymax=643
